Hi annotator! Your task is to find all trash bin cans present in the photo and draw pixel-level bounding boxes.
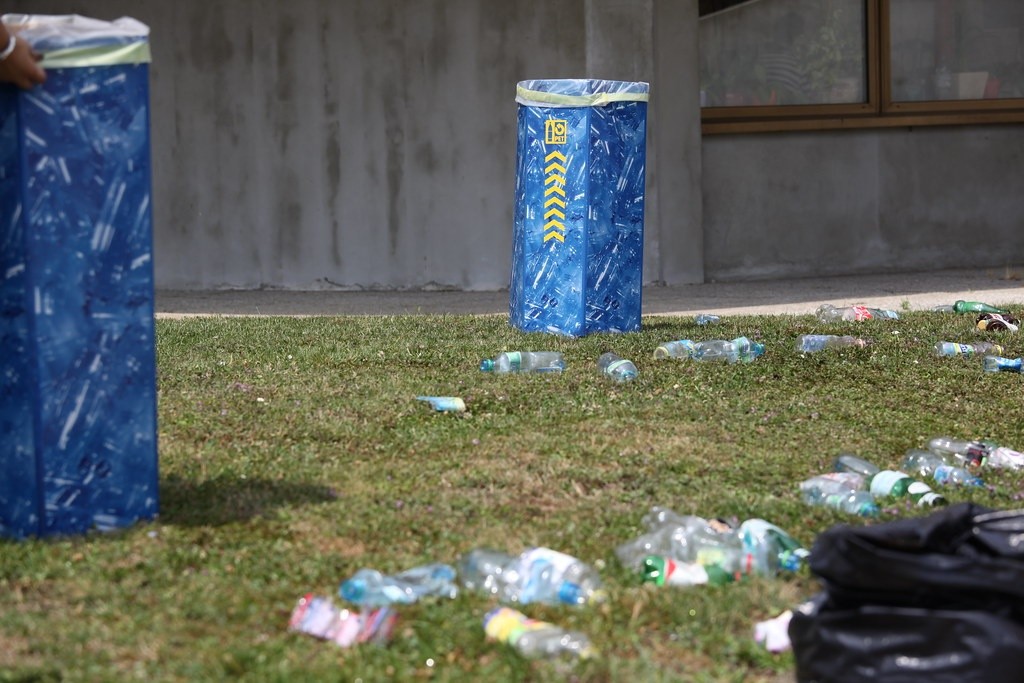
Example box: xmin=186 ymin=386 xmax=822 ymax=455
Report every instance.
xmin=1 ymin=11 xmax=165 ymax=537
xmin=510 ymin=77 xmax=649 ymax=339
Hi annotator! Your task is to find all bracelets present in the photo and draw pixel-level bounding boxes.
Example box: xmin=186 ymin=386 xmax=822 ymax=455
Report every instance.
xmin=0 ymin=35 xmax=16 ymax=60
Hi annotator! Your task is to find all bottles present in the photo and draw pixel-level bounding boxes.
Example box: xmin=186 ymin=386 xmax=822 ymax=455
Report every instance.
xmin=654 ymin=340 xmax=696 ymax=359
xmin=697 ymin=313 xmax=720 ymax=324
xmin=801 ymin=439 xmax=1023 ymax=519
xmin=953 ymin=300 xmax=1010 ymax=316
xmin=481 ymin=352 xmax=566 ymax=374
xmin=614 ymin=507 xmax=812 ymax=588
xmin=796 ymin=335 xmax=865 ymax=353
xmin=815 ymin=304 xmax=890 ymax=324
xmin=693 ymin=337 xmax=764 ymax=364
xmin=976 ymin=314 xmax=1020 ymax=329
xmin=934 ymin=341 xmax=1004 ymax=358
xmin=595 ymin=353 xmax=638 ymax=382
xmin=338 ymin=564 xmax=457 ymax=606
xmin=986 ymin=320 xmax=1008 ymax=340
xmin=983 ymin=355 xmax=1024 ymax=373
xmin=461 ymin=548 xmax=611 ymax=660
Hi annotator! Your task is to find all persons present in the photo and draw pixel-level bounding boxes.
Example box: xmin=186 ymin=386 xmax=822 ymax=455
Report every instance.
xmin=0 ymin=21 xmax=47 ymax=91
